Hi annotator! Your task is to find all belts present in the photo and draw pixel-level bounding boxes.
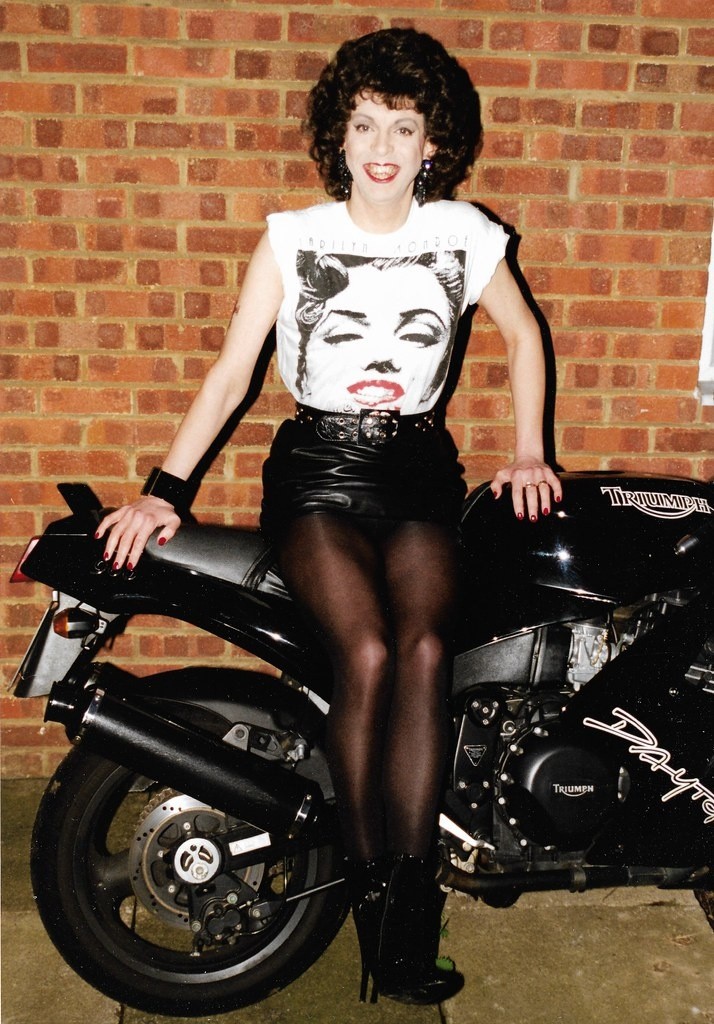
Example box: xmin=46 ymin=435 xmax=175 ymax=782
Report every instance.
xmin=293 ymin=402 xmax=443 ymax=446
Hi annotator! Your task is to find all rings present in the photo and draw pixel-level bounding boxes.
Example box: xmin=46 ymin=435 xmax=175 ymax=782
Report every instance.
xmin=538 ymin=480 xmax=547 ymax=486
xmin=525 ymin=483 xmax=535 ymax=487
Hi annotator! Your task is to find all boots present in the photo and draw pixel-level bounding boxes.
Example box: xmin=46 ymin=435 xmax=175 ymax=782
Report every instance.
xmin=345 ymin=855 xmax=467 ymax=1004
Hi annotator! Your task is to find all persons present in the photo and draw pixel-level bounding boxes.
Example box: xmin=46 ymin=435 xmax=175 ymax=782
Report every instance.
xmin=95 ymin=28 xmax=563 ymax=1005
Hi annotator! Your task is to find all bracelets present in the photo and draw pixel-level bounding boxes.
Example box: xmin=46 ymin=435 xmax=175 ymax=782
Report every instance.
xmin=141 ymin=467 xmax=186 ymax=506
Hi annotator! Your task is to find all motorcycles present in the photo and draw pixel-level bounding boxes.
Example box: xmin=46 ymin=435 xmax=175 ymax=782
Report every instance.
xmin=5 ymin=457 xmax=714 ymax=1022
